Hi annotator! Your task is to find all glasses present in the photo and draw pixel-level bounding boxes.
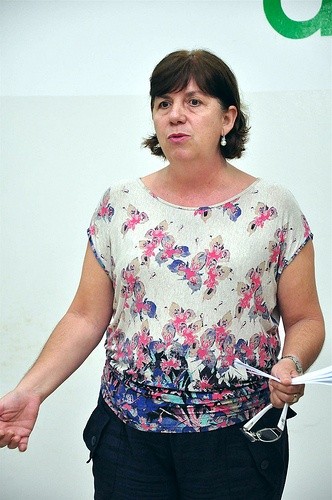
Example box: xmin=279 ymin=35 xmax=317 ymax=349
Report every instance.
xmin=239 ymin=403 xmax=288 ymax=444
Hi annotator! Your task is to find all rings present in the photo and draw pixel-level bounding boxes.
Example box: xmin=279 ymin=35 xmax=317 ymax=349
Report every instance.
xmin=293 ymin=393 xmax=298 ymax=402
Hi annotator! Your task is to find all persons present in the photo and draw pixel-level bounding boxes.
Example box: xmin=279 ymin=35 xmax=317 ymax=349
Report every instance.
xmin=0 ymin=46 xmax=327 ymax=500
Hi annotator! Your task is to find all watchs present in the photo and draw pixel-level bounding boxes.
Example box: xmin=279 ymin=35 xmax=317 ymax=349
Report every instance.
xmin=280 ymin=354 xmax=304 ymax=377
